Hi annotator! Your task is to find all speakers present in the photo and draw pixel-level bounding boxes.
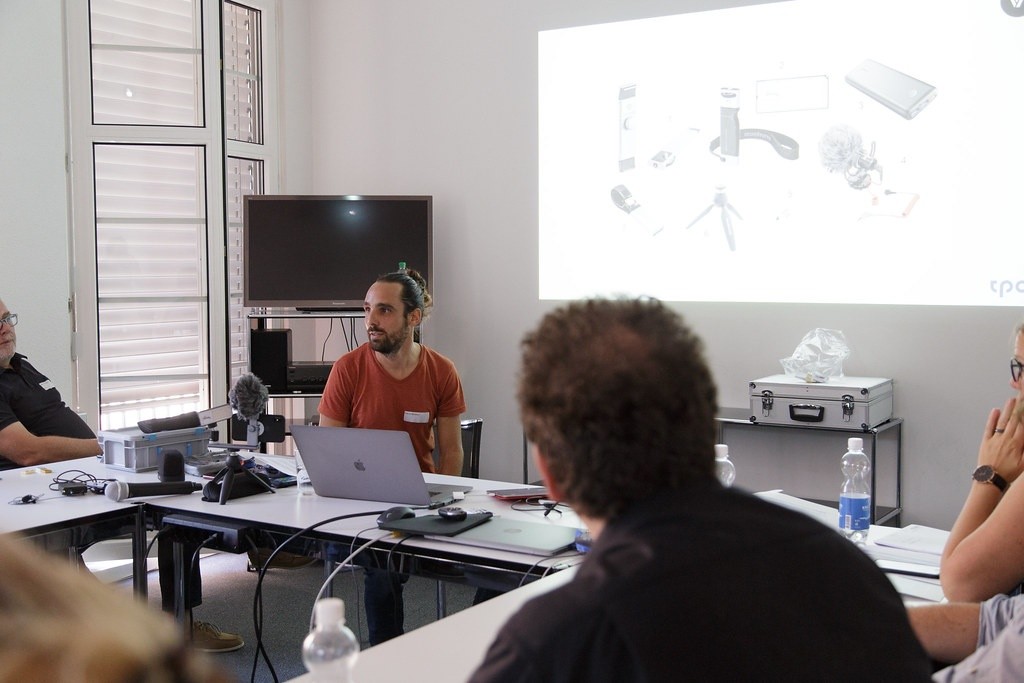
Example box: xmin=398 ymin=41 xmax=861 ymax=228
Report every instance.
xmin=250 ymin=328 xmax=294 ymax=394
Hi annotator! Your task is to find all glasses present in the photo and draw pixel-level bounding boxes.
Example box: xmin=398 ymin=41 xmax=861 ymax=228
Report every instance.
xmin=1010 ymin=357 xmax=1024 ymax=382
xmin=0 ymin=313 xmax=19 ymax=331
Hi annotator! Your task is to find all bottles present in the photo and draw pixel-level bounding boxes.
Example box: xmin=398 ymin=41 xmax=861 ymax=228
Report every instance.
xmin=714 ymin=444 xmax=736 ymax=487
xmin=302 ymin=600 xmax=358 ymax=681
xmin=840 ymin=440 xmax=870 ymax=545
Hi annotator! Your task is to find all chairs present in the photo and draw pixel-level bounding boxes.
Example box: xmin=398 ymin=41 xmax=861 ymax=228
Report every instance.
xmin=324 ymin=417 xmax=484 ymax=624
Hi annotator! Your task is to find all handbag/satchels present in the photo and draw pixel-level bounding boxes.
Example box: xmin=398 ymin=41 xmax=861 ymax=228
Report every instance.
xmin=780 ymin=328 xmax=850 ymax=384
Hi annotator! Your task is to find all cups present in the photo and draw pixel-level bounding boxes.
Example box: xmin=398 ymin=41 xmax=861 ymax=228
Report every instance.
xmin=296 ymin=449 xmax=314 ymax=494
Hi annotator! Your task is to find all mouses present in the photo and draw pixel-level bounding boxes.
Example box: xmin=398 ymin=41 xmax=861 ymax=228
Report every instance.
xmin=376 ymin=507 xmax=416 ymax=530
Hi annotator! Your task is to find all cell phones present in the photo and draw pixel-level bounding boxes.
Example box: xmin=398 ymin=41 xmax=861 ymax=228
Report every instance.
xmin=232 ymin=413 xmax=285 ymax=443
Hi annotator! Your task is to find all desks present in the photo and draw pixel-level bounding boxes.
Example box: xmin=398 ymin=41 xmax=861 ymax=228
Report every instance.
xmin=275 ymin=560 xmax=949 ymax=683
xmin=146 ymin=471 xmax=600 ymax=644
xmin=0 ymin=447 xmax=227 ymax=611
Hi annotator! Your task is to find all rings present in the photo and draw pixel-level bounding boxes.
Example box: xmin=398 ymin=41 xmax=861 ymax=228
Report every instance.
xmin=994 ymin=429 xmax=1004 ymax=432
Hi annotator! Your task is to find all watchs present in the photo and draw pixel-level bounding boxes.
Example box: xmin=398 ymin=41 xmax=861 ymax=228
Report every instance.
xmin=972 ymin=464 xmax=1008 ymax=493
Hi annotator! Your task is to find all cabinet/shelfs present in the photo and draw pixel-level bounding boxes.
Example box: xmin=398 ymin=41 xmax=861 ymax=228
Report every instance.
xmin=246 ymin=312 xmax=423 ymax=454
xmin=521 ymin=405 xmax=905 ymax=529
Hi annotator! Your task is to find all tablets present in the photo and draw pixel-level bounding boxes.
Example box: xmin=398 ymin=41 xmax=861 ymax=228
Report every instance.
xmin=487 ymin=487 xmax=549 ymax=501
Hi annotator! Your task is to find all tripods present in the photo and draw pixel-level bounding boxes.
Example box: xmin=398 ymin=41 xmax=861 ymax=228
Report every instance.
xmin=212 ymin=450 xmax=275 ymax=505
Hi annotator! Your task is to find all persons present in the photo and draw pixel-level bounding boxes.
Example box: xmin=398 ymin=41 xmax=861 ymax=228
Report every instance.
xmin=0 ymin=301 xmax=245 ymax=653
xmin=317 ymin=270 xmax=503 ymax=647
xmin=467 ymin=296 xmax=936 ymax=683
xmin=0 ymin=535 xmax=225 ymax=683
xmin=909 ymin=593 xmax=1024 ymax=683
xmin=939 ymin=321 xmax=1024 ymax=603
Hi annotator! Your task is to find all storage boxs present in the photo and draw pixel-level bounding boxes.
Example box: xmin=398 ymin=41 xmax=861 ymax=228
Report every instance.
xmin=96 ymin=424 xmax=211 ymax=473
xmin=749 ymin=373 xmax=893 ymax=431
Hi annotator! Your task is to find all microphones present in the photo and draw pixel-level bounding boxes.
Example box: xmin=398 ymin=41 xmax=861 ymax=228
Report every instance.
xmin=105 ymin=481 xmax=204 ymax=501
xmin=227 ymin=372 xmax=270 ymax=422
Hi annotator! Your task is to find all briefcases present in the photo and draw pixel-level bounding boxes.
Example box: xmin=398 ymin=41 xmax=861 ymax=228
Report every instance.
xmin=749 ymin=373 xmax=893 ymax=431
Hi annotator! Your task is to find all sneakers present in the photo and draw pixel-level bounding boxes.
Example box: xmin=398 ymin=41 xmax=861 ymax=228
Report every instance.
xmin=183 ymin=616 xmax=244 ymax=652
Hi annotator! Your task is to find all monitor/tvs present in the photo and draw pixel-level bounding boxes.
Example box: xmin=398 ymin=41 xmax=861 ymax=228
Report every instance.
xmin=245 ymin=195 xmax=434 ymax=317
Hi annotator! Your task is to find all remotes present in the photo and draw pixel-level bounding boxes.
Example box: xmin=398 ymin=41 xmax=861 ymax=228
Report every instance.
xmin=438 ymin=507 xmax=468 ymax=521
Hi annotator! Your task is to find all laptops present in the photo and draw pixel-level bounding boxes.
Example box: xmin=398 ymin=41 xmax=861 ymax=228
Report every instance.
xmin=289 ymin=424 xmax=474 ymax=506
